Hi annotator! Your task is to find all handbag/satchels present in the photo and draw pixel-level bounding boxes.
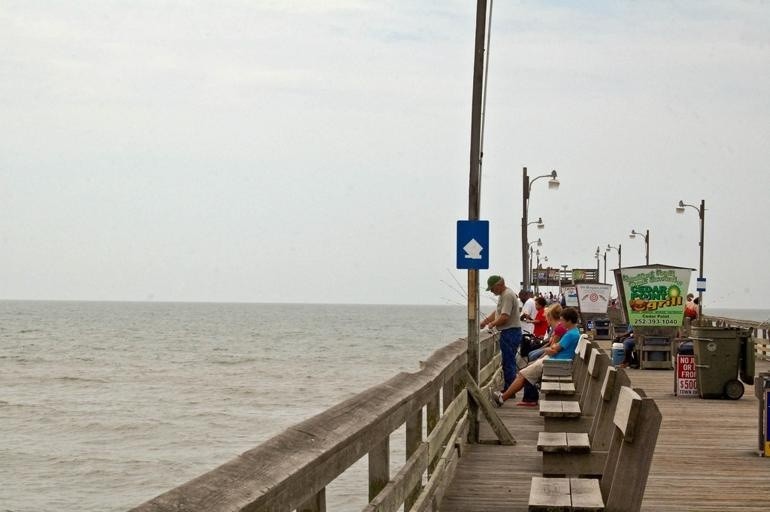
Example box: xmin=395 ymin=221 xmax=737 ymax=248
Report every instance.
xmin=521 ymin=333 xmax=549 ymax=356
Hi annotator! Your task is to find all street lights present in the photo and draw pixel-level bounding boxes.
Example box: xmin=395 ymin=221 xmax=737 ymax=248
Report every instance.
xmin=606 ymin=244 xmax=621 ymax=268
xmin=561 ymin=265 xmax=568 ymax=277
xmin=629 ymin=229 xmax=649 ymax=265
xmin=594 ymin=252 xmax=606 ymax=284
xmin=675 ymin=199 xmax=705 ymax=318
xmin=521 ymin=167 xmax=560 ymax=295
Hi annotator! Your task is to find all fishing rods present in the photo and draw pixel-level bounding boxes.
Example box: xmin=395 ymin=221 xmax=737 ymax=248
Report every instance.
xmin=441 ymin=268 xmax=529 ymax=370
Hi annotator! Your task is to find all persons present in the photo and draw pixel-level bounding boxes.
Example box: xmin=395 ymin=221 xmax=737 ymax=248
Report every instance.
xmin=611 ymin=322 xmax=635 ymax=369
xmin=684 ymin=297 xmax=700 ymax=320
xmin=687 ymin=293 xmax=694 ymax=302
xmin=480 ymin=275 xmax=581 ymax=406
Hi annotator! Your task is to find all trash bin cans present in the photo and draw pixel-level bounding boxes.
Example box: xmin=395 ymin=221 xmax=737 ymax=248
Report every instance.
xmin=688 ymin=326 xmax=755 ymax=400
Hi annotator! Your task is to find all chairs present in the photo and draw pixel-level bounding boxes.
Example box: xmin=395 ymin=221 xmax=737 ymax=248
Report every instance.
xmin=527 ymin=333 xmax=662 ymax=512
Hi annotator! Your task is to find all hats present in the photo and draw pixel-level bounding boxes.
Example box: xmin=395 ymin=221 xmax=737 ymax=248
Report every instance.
xmin=486 ymin=275 xmax=501 ymax=291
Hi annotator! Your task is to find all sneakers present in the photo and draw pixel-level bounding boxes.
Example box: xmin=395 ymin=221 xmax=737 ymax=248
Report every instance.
xmin=493 ymin=390 xmax=538 ymax=406
